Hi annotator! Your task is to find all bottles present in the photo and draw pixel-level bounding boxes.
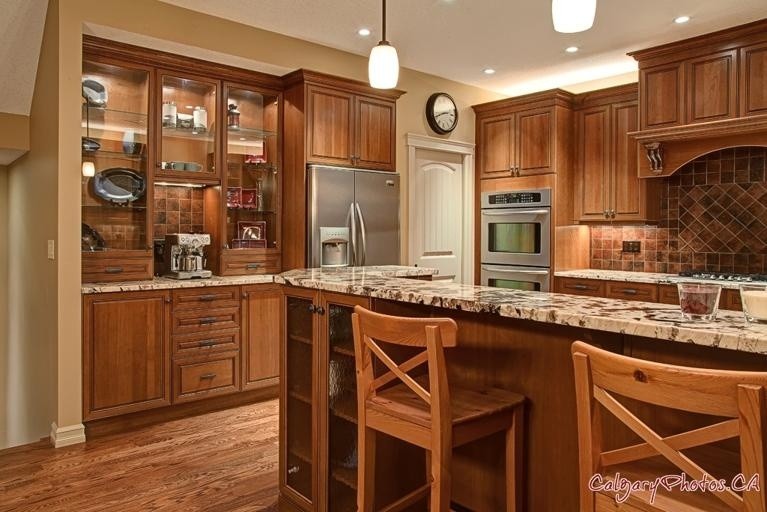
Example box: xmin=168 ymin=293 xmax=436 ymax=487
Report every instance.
xmin=192 ymin=106 xmax=207 ymax=131
xmin=227 ymin=104 xmax=240 ymax=128
xmin=162 ymin=102 xmax=177 ymax=128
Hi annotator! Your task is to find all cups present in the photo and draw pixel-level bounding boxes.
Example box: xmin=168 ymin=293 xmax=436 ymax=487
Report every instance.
xmin=170 ymin=161 xmax=204 ymax=172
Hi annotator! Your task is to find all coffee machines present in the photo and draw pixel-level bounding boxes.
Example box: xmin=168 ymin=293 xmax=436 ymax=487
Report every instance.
xmin=164 ymin=232 xmax=211 ymax=280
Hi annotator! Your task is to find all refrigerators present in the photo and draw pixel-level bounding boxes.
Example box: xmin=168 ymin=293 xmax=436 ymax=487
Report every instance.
xmin=307 ymin=164 xmax=401 ymax=268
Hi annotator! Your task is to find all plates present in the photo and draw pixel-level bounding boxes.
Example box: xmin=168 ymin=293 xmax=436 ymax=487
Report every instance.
xmin=88 ymin=168 xmax=146 ymax=204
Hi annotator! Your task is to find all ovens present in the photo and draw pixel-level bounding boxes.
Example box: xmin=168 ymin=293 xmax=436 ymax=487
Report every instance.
xmin=480 ymin=188 xmax=550 ymax=267
xmin=480 ymin=264 xmax=550 ymax=292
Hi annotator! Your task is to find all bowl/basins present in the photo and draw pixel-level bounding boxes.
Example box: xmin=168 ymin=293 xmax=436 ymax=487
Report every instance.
xmin=121 ymin=140 xmax=145 ymax=158
xmin=676 ymin=284 xmax=722 ymax=320
xmin=740 ymin=284 xmax=767 ymax=323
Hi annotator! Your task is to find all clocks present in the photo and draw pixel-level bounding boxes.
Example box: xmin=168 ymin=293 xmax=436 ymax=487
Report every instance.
xmin=425 ymin=92 xmax=459 ymax=134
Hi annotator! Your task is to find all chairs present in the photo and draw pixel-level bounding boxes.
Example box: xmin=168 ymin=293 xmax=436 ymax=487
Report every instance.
xmin=349 ymin=304 xmax=527 ymax=512
xmin=570 ymin=341 xmax=767 ymax=512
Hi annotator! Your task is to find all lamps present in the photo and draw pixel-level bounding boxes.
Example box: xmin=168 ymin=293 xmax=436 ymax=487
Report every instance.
xmin=551 ymin=0 xmax=597 ymax=34
xmin=368 ymin=0 xmax=400 ymax=89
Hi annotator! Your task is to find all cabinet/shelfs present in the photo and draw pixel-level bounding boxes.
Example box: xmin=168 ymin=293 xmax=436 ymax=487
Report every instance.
xmin=203 ymin=60 xmax=284 ymax=276
xmin=242 ymin=283 xmax=281 ymax=406
xmin=82 ymin=291 xmax=171 ymax=438
xmin=472 ymin=87 xmax=574 ymax=182
xmin=153 ymin=50 xmax=222 ymax=189
xmin=625 ymin=29 xmax=767 ymax=141
xmin=555 ymin=277 xmax=744 ymax=313
xmin=170 ymin=285 xmax=241 ymax=422
xmin=575 ymin=82 xmax=660 ymax=222
xmin=277 ymin=285 xmax=369 ymax=512
xmin=283 ymin=69 xmax=406 ymax=171
xmin=81 ymin=31 xmax=154 ymax=283
